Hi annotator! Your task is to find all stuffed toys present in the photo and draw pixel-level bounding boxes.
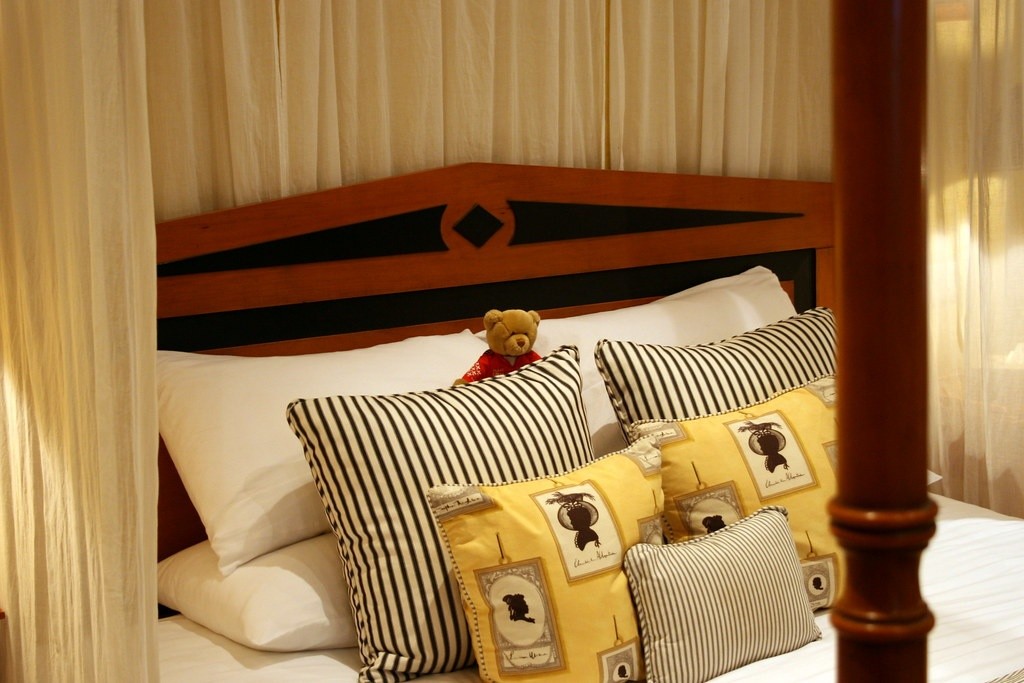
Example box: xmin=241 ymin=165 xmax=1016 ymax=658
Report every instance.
xmin=450 ymin=309 xmax=541 ymax=386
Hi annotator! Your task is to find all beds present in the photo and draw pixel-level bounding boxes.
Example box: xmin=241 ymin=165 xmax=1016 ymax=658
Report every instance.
xmin=154 ymin=162 xmax=1024 ymax=682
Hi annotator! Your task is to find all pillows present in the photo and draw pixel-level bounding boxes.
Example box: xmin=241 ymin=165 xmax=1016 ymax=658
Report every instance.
xmin=157 ymin=531 xmax=359 ymax=653
xmin=628 ymin=374 xmax=846 ymax=613
xmin=595 ymin=306 xmax=840 ymax=447
xmin=474 ymin=266 xmax=798 ymax=459
xmin=285 ymin=345 xmax=595 ymax=683
xmin=156 ymin=328 xmax=490 ymax=576
xmin=426 ymin=435 xmax=664 ymax=683
xmin=623 ymin=506 xmax=823 ymax=683
